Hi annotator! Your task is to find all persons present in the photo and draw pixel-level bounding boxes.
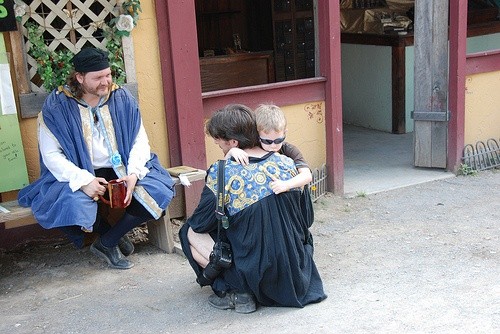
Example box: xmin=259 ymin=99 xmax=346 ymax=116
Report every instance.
xmin=18 ymin=47 xmax=175 ymax=268
xmin=179 ymin=104 xmax=326 ymax=314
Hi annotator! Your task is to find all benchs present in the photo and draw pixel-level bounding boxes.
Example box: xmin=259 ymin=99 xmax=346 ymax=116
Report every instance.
xmin=0 ymin=165 xmax=208 ymax=254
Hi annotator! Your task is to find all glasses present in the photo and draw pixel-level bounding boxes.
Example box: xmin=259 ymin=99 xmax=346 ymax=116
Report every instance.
xmin=258 ymin=136 xmax=286 ymax=145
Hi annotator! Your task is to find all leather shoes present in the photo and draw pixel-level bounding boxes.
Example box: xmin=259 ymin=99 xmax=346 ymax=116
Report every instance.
xmin=90 ymin=238 xmax=134 ymax=268
xmin=208 ymin=291 xmax=256 ymax=313
xmin=118 ymin=234 xmax=134 ymax=256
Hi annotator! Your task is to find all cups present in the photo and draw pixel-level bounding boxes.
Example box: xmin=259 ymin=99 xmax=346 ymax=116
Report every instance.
xmin=98 ymin=179 xmax=126 ymax=209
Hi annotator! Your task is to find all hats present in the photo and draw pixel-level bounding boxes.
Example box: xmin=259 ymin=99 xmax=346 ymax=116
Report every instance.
xmin=74 ymin=47 xmax=110 ymax=71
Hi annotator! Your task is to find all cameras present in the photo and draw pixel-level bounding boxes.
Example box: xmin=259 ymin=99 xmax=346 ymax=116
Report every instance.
xmin=196 ymin=242 xmax=233 ymax=287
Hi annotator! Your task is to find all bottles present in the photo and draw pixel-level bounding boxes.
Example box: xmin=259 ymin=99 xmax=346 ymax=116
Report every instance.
xmin=233 ymin=33 xmax=241 ymax=51
